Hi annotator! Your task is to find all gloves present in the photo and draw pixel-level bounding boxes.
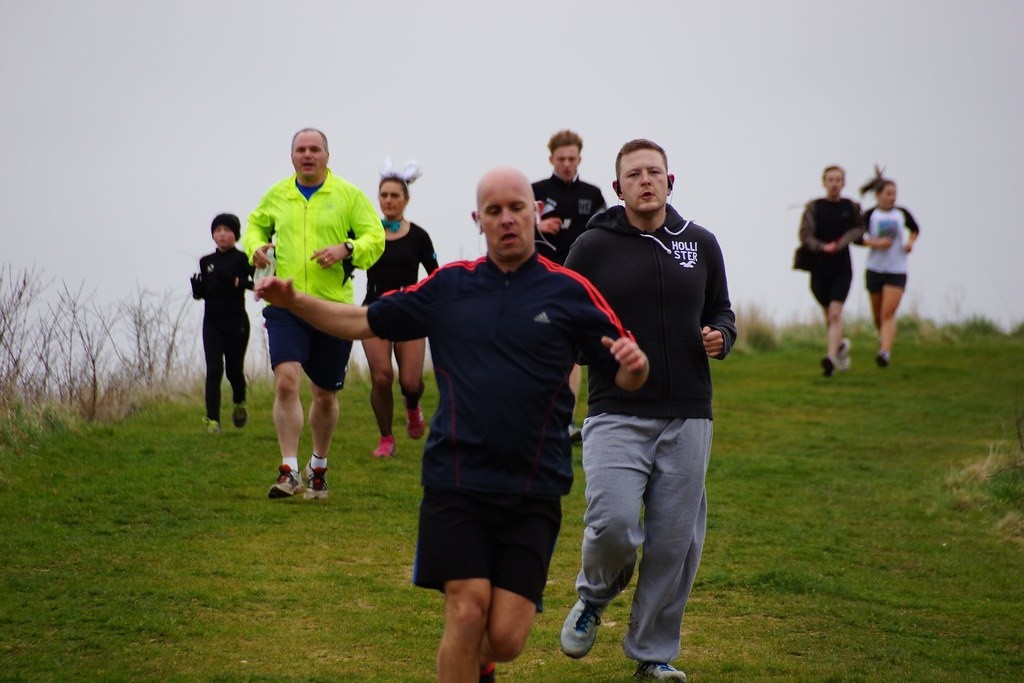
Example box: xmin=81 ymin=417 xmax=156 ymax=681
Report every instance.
xmin=190 ymin=272 xmax=202 ymax=299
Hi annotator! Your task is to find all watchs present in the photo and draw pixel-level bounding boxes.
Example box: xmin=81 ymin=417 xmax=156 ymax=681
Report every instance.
xmin=345 ymin=242 xmax=354 ymax=252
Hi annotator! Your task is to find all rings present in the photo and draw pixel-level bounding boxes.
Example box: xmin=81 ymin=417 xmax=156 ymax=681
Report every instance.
xmin=325 ymin=258 xmax=328 ymax=263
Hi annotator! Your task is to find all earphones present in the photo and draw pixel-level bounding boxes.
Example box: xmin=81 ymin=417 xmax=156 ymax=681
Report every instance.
xmin=667 ymin=176 xmax=672 ymax=191
xmin=477 ymin=219 xmax=482 ymax=235
xmin=533 ymin=211 xmax=540 ymax=227
xmin=615 ymin=180 xmax=622 ymax=196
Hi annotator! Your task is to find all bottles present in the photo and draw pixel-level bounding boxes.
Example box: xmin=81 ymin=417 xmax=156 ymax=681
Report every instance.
xmin=254 ymin=247 xmax=276 ymax=284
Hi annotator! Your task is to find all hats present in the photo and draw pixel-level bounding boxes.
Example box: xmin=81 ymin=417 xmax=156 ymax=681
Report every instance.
xmin=211 ymin=214 xmax=241 ymax=242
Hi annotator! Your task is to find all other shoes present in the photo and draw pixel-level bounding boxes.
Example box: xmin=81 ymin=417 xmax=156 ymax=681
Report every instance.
xmin=835 ymin=339 xmax=851 ymax=370
xmin=568 ymin=424 xmax=582 ymax=442
xmin=821 ymin=356 xmax=835 ymax=376
xmin=875 ymin=352 xmax=890 ymax=368
xmin=480 ymin=657 xmax=498 ymax=683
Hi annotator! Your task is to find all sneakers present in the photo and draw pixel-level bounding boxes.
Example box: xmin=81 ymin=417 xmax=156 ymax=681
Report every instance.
xmin=268 ymin=464 xmax=305 ymax=498
xmin=631 ymin=657 xmax=687 ymax=683
xmin=202 ymin=416 xmax=220 ymax=434
xmin=373 ymin=436 xmax=398 ymax=459
xmin=404 ymin=398 xmax=426 ymax=438
xmin=560 ymin=596 xmax=610 ymax=659
xmin=303 ymin=459 xmax=329 ymax=499
xmin=232 ymin=399 xmax=248 ymax=429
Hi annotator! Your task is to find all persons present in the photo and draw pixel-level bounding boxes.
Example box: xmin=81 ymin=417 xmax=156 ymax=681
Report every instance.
xmin=853 ymin=179 xmax=920 ymax=368
xmin=190 ymin=213 xmax=256 ymax=434
xmin=564 ymin=139 xmax=738 ymax=683
xmin=530 ymin=130 xmax=607 ymax=440
xmin=798 ymin=165 xmax=866 ymax=378
xmin=253 ymin=166 xmax=649 ymax=683
xmin=360 ymin=174 xmax=439 ymax=458
xmin=242 ymin=129 xmax=386 ymax=502
